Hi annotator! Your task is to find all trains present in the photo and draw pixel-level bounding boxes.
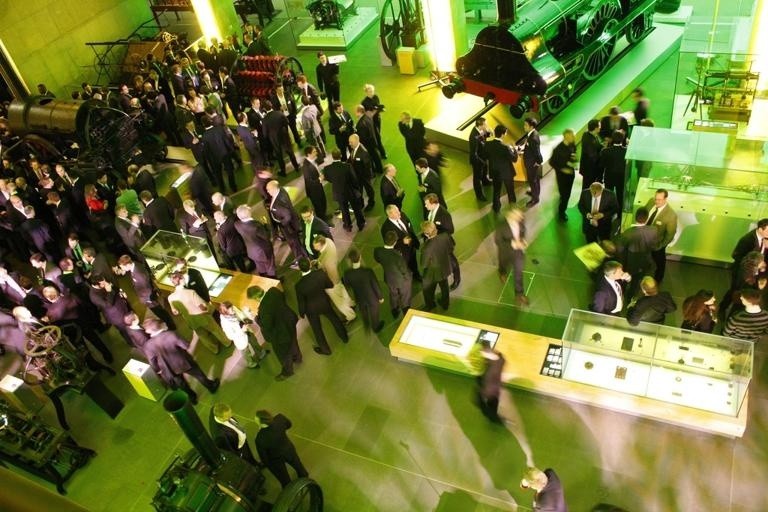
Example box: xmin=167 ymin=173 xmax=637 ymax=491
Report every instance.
xmin=415 ymin=1 xmax=683 ymax=119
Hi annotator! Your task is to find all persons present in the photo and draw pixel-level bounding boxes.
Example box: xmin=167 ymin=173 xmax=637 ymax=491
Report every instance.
xmin=0 ymin=21 xmax=768 ymax=512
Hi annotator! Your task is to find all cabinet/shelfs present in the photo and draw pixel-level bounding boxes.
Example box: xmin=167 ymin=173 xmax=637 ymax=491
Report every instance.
xmin=619 ymin=123 xmax=767 ymax=269
xmin=283 ymin=0 xmax=381 ymax=50
xmin=141 ymin=230 xmax=278 ymax=321
xmin=389 ymin=306 xmax=752 ymax=440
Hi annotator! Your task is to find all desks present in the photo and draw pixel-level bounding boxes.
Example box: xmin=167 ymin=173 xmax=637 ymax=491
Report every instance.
xmin=1 ymin=412 xmax=95 ymax=496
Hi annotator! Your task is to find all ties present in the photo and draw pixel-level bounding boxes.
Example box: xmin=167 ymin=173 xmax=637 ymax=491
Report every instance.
xmin=398 ymin=221 xmax=407 ymax=235
xmin=229 ymin=419 xmax=246 ymax=434
xmin=646 ymin=207 xmax=658 ymax=226
xmin=615 ymin=283 xmax=622 ymax=302
xmin=340 ymin=114 xmax=347 ymax=125
xmin=592 ymin=200 xmax=598 ymax=227
